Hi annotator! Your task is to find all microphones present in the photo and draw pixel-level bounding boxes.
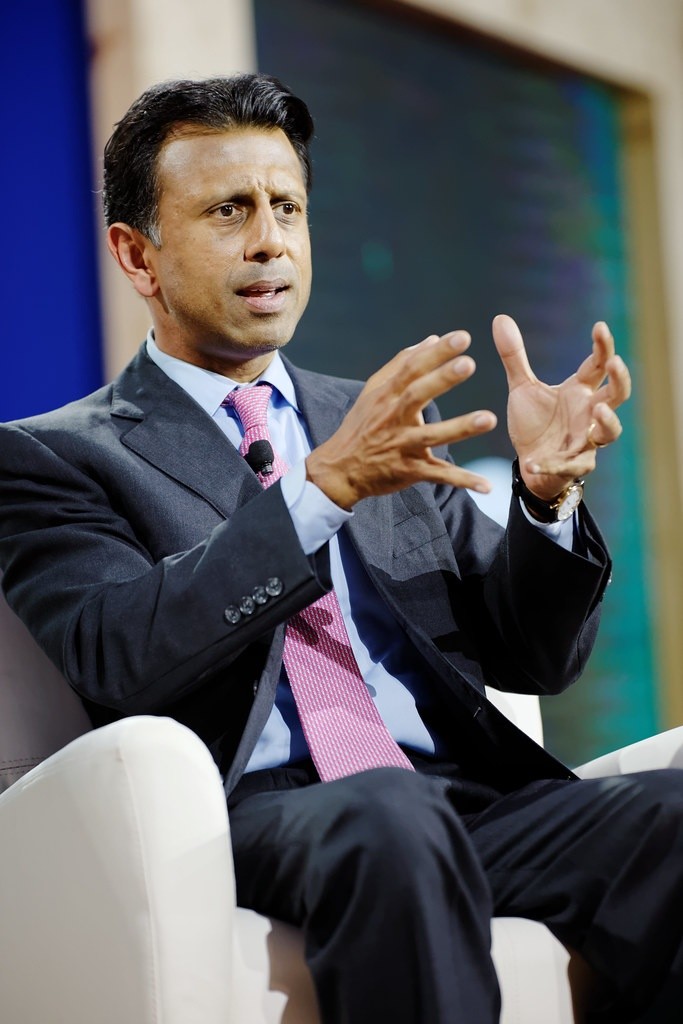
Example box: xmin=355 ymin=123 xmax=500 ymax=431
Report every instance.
xmin=249 ymin=439 xmax=273 ymax=476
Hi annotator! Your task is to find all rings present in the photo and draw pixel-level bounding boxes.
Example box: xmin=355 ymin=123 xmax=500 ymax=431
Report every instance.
xmin=588 ymin=424 xmax=607 ymax=448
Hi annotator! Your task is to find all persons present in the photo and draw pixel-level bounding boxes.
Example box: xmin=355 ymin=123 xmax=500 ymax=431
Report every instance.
xmin=0 ymin=73 xmax=683 ymax=1023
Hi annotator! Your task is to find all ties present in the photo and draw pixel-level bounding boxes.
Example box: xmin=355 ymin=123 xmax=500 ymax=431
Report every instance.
xmin=220 ymin=382 xmax=416 ymax=784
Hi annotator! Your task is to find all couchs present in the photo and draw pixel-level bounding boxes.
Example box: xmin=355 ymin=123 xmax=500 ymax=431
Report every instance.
xmin=0 ymin=458 xmax=683 ymax=1024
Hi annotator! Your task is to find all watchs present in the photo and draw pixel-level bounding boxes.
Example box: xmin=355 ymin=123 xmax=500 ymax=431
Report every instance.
xmin=512 ymin=456 xmax=585 ymax=523
